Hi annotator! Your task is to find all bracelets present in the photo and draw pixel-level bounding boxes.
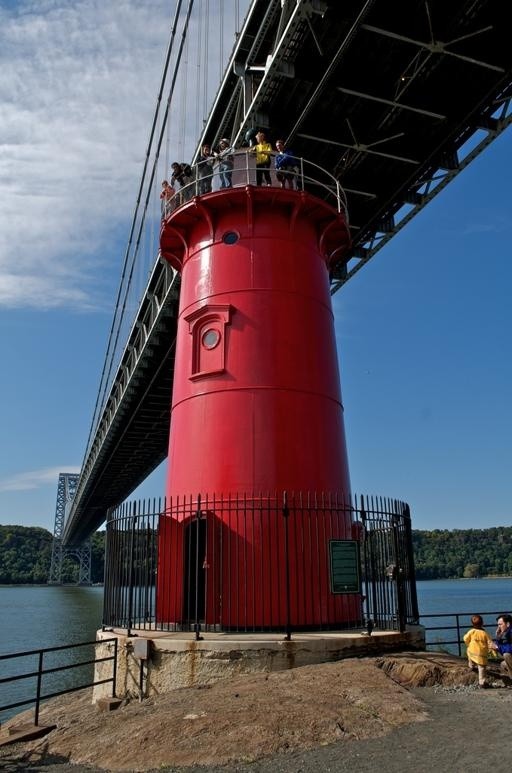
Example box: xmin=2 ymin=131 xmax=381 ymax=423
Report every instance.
xmin=495 ymin=644 xmax=499 ymax=650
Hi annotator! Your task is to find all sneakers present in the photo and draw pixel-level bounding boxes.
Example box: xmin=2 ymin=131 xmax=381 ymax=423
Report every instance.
xmin=481 ymin=682 xmax=490 ymax=689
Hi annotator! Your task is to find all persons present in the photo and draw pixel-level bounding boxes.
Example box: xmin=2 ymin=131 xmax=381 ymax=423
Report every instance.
xmin=486 ymin=614 xmax=512 ymax=680
xmin=275 ymin=138 xmax=298 ymax=189
xmin=217 ymin=137 xmax=235 ymax=189
xmin=250 ymin=130 xmax=272 ymax=186
xmin=195 ymin=143 xmax=215 ymax=193
xmin=463 ymin=614 xmax=498 ymax=688
xmin=159 ymin=179 xmax=177 ymax=207
xmin=169 ymin=161 xmax=193 ymax=201
